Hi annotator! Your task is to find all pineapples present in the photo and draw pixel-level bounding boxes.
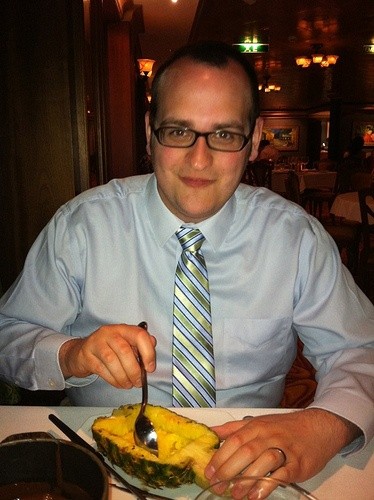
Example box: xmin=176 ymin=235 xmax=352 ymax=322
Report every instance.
xmin=92 ymin=403 xmax=243 ymax=496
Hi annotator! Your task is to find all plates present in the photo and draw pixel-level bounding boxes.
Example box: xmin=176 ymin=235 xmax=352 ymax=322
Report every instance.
xmin=72 ymin=413 xmax=215 ymax=500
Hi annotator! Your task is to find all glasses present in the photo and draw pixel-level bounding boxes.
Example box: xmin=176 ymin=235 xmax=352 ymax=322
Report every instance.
xmin=151 ymin=123 xmax=253 ymax=151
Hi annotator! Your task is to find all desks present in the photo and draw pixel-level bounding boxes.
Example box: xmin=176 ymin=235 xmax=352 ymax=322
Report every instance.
xmin=301 ymin=166 xmax=336 ymax=191
xmin=0 ymin=405 xmax=373 ymax=500
xmin=267 ymin=168 xmax=313 ymax=193
xmin=329 ymin=190 xmax=374 ymax=233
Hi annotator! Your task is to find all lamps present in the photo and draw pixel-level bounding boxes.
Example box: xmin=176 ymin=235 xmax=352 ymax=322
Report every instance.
xmin=138 ymin=55 xmax=155 ymax=82
xmin=258 ymin=75 xmax=283 ymax=92
xmin=295 ymin=43 xmax=340 ymax=69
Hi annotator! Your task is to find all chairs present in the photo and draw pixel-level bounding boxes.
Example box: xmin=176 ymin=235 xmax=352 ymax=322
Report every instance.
xmin=249 ymin=159 xmax=374 ymax=305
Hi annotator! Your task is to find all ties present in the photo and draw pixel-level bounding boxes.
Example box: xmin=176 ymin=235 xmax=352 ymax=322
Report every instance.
xmin=172 ymin=226 xmax=216 ymax=408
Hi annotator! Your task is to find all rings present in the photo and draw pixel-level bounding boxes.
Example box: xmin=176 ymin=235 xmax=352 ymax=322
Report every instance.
xmin=269 ymin=447 xmax=288 ymax=464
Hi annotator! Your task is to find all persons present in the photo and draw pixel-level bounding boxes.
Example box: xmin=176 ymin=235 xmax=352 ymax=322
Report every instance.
xmin=0 ymin=42 xmax=374 ymax=500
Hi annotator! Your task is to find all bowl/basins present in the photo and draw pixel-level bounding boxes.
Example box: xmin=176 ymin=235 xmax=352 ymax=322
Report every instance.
xmin=0 ymin=431 xmax=111 ymax=500
xmin=194 ymin=475 xmax=319 ymax=500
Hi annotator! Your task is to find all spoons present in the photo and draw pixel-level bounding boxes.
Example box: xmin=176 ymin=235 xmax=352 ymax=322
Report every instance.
xmin=134 ymin=321 xmax=162 ymax=456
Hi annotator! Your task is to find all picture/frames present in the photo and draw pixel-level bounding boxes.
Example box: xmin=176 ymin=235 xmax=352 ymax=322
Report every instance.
xmin=262 ymin=125 xmax=300 ymax=151
